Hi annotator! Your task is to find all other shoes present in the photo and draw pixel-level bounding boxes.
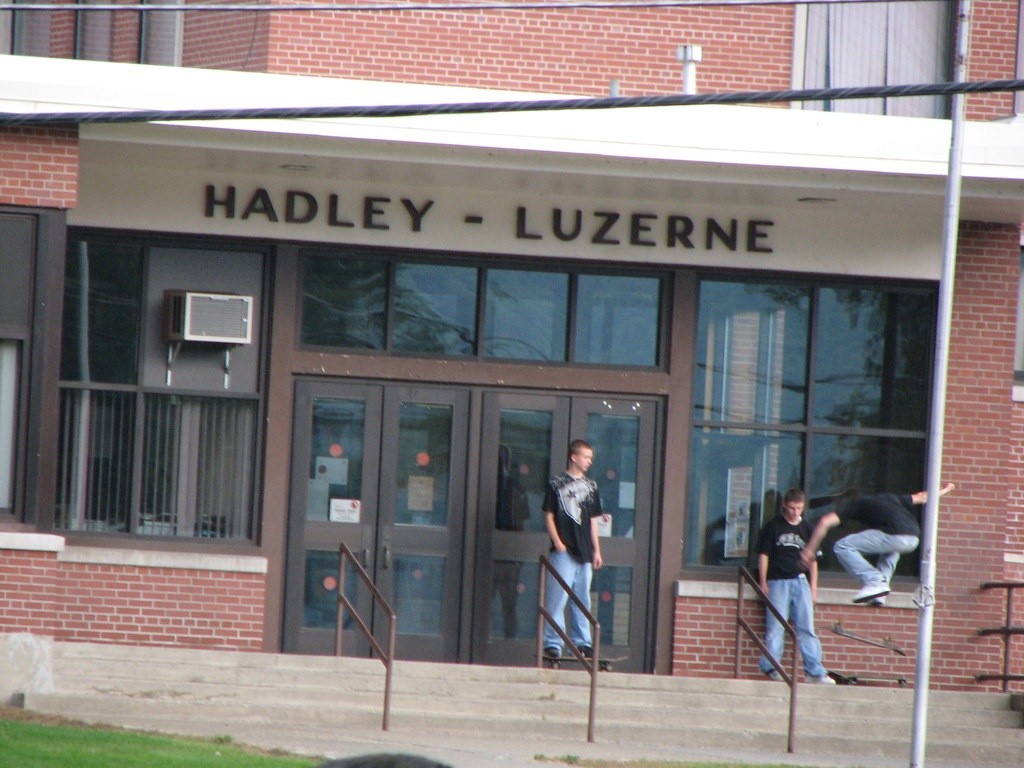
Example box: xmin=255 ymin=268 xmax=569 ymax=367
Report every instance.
xmin=852 ymin=577 xmax=890 ymax=603
xmin=869 ymin=596 xmax=887 ymax=608
xmin=577 ymin=646 xmax=594 ymax=658
xmin=545 ymin=648 xmax=562 ymax=658
xmin=767 ymin=670 xmax=786 ymax=681
xmin=803 ymin=671 xmax=836 ymax=686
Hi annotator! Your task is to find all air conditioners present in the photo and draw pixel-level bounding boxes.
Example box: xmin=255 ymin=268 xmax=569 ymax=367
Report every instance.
xmin=164 ymin=289 xmax=254 ymax=345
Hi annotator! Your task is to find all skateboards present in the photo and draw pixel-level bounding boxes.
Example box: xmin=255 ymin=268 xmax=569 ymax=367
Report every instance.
xmin=530 ymin=650 xmax=630 ymax=671
xmin=818 ymin=619 xmax=908 ymax=657
xmin=830 ymin=669 xmax=910 ymax=686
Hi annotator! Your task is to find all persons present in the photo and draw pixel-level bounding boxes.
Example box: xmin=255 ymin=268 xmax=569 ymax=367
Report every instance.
xmin=542 ymin=441 xmax=604 ymax=655
xmin=485 ymin=444 xmax=530 ymax=638
xmin=758 ymin=487 xmax=840 ymax=685
xmin=799 ymin=483 xmax=957 ymax=605
